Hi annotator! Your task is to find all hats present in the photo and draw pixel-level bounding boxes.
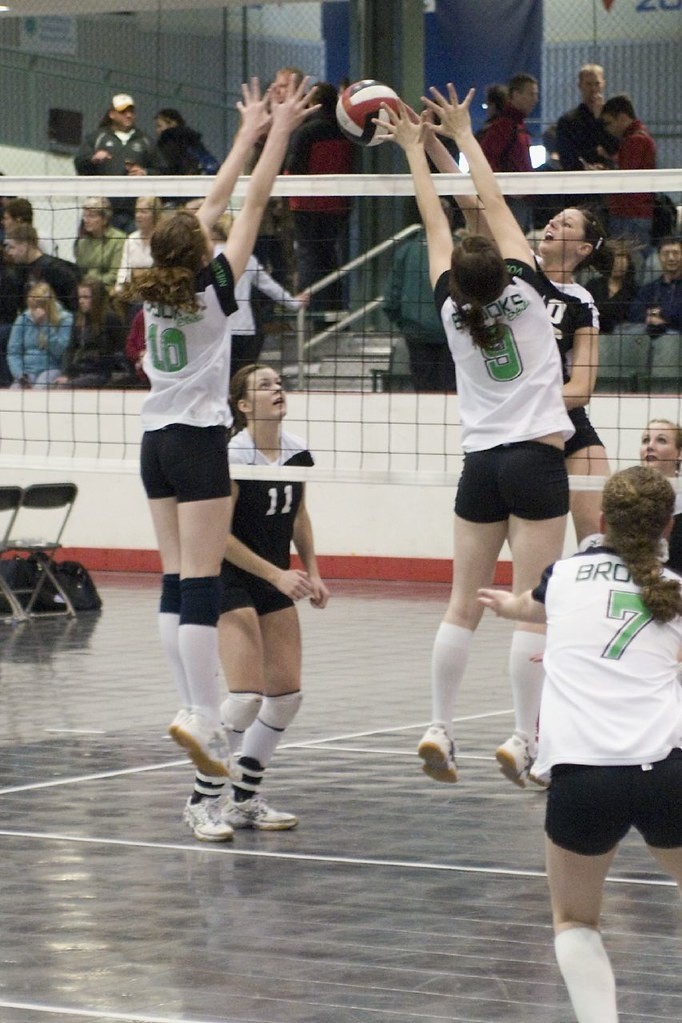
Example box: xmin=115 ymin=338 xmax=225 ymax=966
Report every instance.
xmin=153 ymin=108 xmax=184 ymax=128
xmin=110 ymin=93 xmax=135 ymax=112
xmin=80 ymin=196 xmax=112 ymax=218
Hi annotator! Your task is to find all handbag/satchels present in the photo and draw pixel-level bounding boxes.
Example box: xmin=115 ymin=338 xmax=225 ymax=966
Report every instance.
xmin=0 ymin=547 xmax=101 ymax=613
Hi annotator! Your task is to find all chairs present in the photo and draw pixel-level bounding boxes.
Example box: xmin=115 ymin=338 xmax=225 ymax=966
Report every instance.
xmin=0 ymin=482 xmax=79 ymax=622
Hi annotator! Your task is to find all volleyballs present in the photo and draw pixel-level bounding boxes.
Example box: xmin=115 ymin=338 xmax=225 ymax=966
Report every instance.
xmin=335 ymin=80 xmax=400 ymax=146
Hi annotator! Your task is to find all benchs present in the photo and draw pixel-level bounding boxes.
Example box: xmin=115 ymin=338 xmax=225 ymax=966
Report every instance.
xmin=270 ymin=308 xmax=348 ymax=322
xmin=367 ymin=331 xmax=681 ymax=393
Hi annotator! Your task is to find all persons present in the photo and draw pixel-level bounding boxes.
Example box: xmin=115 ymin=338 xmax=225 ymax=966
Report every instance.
xmin=477 ymin=466 xmax=682 ymax=1023
xmin=138 ymin=74 xmax=321 ymax=779
xmin=393 ymin=97 xmax=612 ymax=547
xmin=371 ymin=82 xmax=570 ymax=788
xmin=641 ymin=419 xmax=682 ymax=579
xmin=0 ymin=62 xmax=682 ymax=395
xmin=179 ymin=364 xmax=330 ymax=841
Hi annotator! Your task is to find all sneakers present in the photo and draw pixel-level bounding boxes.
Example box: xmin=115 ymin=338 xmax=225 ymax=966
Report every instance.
xmin=175 ymin=714 xmax=230 ymax=777
xmin=417 ymin=723 xmax=459 ymax=783
xmin=168 ymin=710 xmax=191 ymax=747
xmin=182 ymin=796 xmax=234 ymax=841
xmin=220 ymin=794 xmax=298 ymax=829
xmin=495 ymin=735 xmax=538 ymax=789
